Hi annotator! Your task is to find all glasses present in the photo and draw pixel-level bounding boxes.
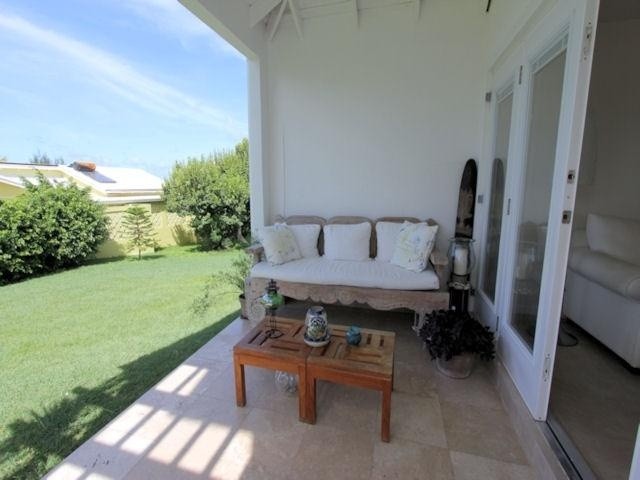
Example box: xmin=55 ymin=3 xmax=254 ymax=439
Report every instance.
xmin=445 ymin=237 xmax=478 ymax=312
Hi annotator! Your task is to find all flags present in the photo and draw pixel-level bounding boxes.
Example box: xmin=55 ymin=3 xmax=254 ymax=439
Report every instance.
xmin=304 ymin=305 xmax=329 ymax=342
xmin=344 ymin=325 xmax=363 ymax=346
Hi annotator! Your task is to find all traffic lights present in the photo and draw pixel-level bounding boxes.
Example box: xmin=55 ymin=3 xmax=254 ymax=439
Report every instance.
xmin=255 ymin=221 xmax=304 ymax=266
xmin=388 ymin=218 xmax=441 ymax=273
xmin=277 ymin=223 xmax=322 ymax=258
xmin=584 ymin=211 xmax=640 ymax=267
xmin=321 ymin=221 xmax=372 ymax=263
xmin=373 ymin=221 xmax=431 ymax=265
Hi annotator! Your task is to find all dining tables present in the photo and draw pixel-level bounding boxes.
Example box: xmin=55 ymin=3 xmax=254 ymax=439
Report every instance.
xmin=419 ymin=305 xmax=498 ymax=382
xmin=234 ymin=261 xmax=253 ymax=320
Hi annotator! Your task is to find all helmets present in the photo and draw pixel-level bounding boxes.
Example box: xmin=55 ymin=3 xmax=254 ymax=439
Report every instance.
xmin=303 ymin=333 xmax=332 ymax=346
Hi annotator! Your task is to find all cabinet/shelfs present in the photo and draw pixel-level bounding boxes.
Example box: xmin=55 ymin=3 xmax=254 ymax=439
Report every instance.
xmin=345 ymin=326 xmax=361 ymax=346
xmin=305 ymin=305 xmax=327 ymax=340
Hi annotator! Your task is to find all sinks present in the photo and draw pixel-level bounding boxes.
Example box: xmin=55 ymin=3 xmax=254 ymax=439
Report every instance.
xmin=242 ymin=214 xmax=451 ymax=334
xmin=560 ymin=210 xmax=640 ymax=369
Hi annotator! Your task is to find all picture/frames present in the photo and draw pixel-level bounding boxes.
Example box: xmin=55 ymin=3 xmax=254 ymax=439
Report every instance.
xmin=230 ymin=314 xmax=325 ymax=423
xmin=304 ymin=322 xmax=397 ymax=443
